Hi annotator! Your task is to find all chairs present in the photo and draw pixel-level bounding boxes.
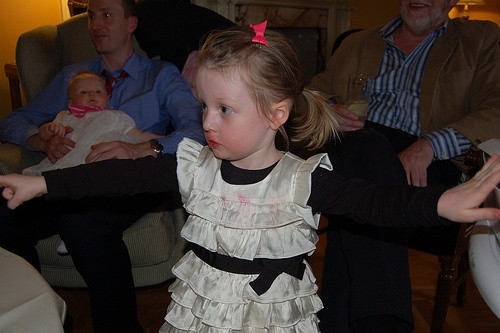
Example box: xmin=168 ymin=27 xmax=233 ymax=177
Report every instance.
xmin=320 ymin=28 xmax=468 ymax=333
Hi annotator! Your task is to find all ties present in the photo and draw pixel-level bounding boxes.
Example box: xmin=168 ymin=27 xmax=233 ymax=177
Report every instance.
xmin=104 ymin=70 xmax=128 ymax=101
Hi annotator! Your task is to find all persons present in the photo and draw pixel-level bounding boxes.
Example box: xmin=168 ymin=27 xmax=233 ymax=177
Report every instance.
xmin=305 ymin=0 xmax=500 ymax=333
xmin=22 ymin=73 xmax=166 ymax=253
xmin=0 ymin=0 xmax=207 ymax=333
xmin=0 ymin=21 xmax=500 ymax=333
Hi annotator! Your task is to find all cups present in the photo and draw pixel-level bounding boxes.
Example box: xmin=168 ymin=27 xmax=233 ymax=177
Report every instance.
xmin=344 ymin=72 xmax=370 ymax=133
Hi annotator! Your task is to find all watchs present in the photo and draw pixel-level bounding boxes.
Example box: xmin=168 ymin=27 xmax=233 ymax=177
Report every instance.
xmin=147 ymin=139 xmax=163 ymax=157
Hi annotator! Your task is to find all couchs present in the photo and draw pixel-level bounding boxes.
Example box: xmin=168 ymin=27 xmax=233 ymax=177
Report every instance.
xmin=0 ymin=0 xmax=238 ymax=289
